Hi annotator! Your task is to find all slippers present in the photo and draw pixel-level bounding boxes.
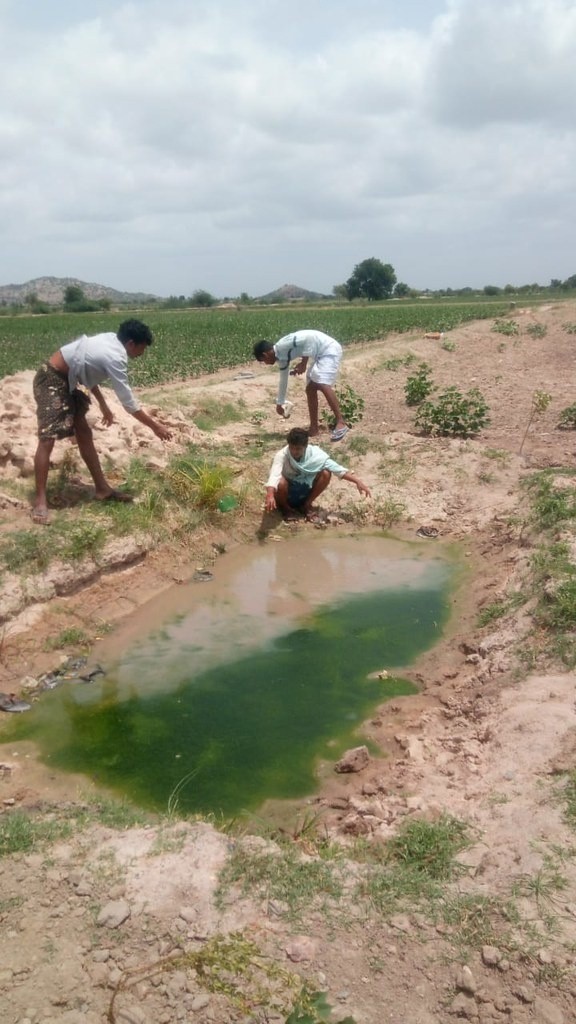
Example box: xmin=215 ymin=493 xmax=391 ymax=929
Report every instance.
xmin=93 ymin=491 xmax=133 ymax=502
xmin=30 ymin=508 xmax=52 ymax=525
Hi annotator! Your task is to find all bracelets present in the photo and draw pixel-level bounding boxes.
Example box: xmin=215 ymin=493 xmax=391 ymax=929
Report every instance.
xmin=266 ymin=493 xmax=273 ymax=498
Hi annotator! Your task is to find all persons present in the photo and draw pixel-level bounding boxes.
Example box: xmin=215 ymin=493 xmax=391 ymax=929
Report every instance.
xmin=32 ymin=321 xmax=172 ymax=527
xmin=260 ymin=427 xmax=371 ymax=522
xmin=254 ymin=328 xmax=352 ymax=442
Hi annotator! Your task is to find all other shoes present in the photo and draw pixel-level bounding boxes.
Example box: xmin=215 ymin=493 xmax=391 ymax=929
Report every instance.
xmin=330 ymin=425 xmax=349 ymax=440
